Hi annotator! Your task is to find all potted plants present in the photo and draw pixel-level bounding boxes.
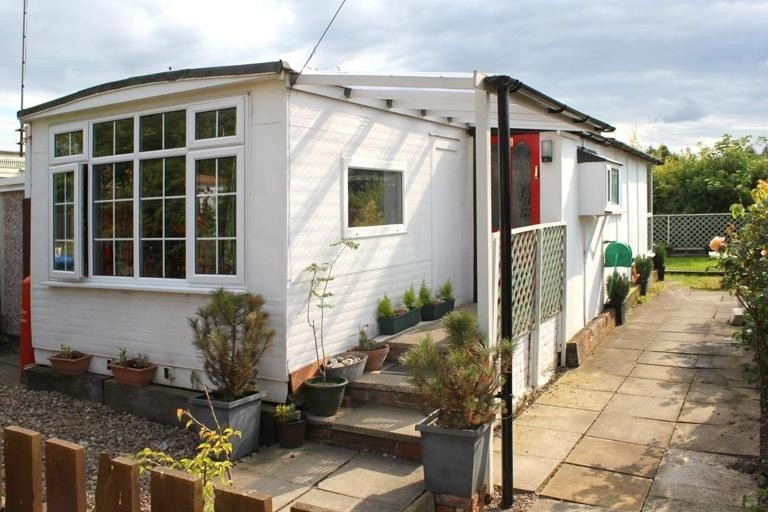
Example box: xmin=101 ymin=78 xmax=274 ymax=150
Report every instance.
xmin=374 ymin=280 xmax=425 ymax=335
xmin=633 ymin=253 xmax=652 ymax=296
xmin=187 ymin=287 xmax=278 ymax=463
xmin=107 ymin=344 xmax=157 ymax=388
xmin=275 ymin=403 xmax=309 ymax=450
xmin=321 ymin=349 xmax=370 ymax=382
xmin=394 ymin=307 xmax=516 ymax=496
xmin=664 ymin=241 xmax=673 ymax=257
xmin=291 ymin=234 xmax=363 ymax=415
xmin=344 ymin=321 xmax=392 ymax=371
xmin=604 ymin=271 xmax=630 ymax=325
xmin=46 ymin=344 xmax=94 ymax=377
xmin=420 ymin=276 xmax=457 ymax=321
xmin=653 ymin=243 xmax=667 ymax=281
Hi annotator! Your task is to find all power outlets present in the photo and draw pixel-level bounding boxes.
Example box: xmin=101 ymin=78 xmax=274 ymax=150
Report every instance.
xmin=164 ymin=367 xmax=174 ymax=380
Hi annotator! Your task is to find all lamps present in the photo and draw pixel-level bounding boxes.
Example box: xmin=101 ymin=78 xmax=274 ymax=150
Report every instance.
xmin=541 ymin=140 xmax=553 ymax=162
